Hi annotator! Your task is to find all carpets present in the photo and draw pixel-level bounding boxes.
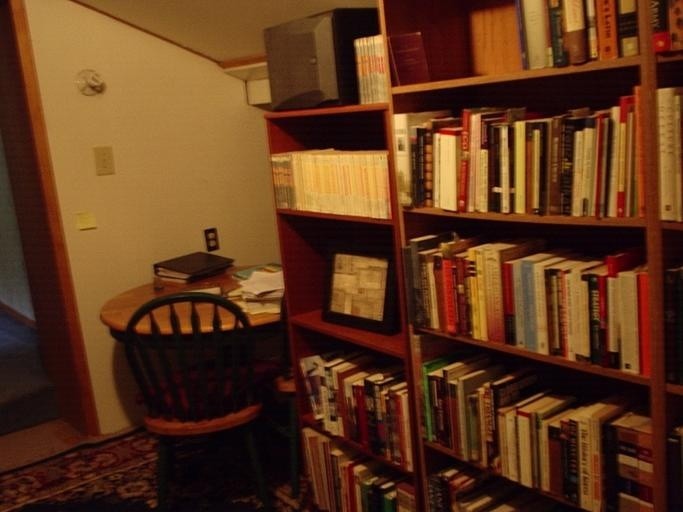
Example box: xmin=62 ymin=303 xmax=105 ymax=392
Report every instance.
xmin=0 ymin=425 xmax=313 ymax=511
xmin=0 ymin=305 xmax=60 ymax=438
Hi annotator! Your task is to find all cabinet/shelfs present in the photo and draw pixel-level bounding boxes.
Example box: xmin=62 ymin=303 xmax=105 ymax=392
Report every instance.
xmin=266 ymin=104 xmax=419 ymax=510
xmin=382 ymin=1 xmax=682 ymax=511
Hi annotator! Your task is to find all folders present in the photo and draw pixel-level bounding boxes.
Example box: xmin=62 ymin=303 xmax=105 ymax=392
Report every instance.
xmin=153 ymin=251 xmax=233 ymax=280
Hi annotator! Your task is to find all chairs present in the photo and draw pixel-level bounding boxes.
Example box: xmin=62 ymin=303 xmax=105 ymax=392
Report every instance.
xmin=123 ymin=292 xmax=275 ymax=512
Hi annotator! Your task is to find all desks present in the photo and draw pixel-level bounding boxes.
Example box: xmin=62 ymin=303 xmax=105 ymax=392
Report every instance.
xmin=99 ymin=264 xmax=300 ymax=498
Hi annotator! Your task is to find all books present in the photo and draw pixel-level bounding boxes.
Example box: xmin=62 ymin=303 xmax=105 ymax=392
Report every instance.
xmin=153 ymin=252 xmax=233 ymax=284
xmin=232 ymin=263 xmax=281 ymax=281
xmin=242 ymin=291 xmax=284 ymax=302
